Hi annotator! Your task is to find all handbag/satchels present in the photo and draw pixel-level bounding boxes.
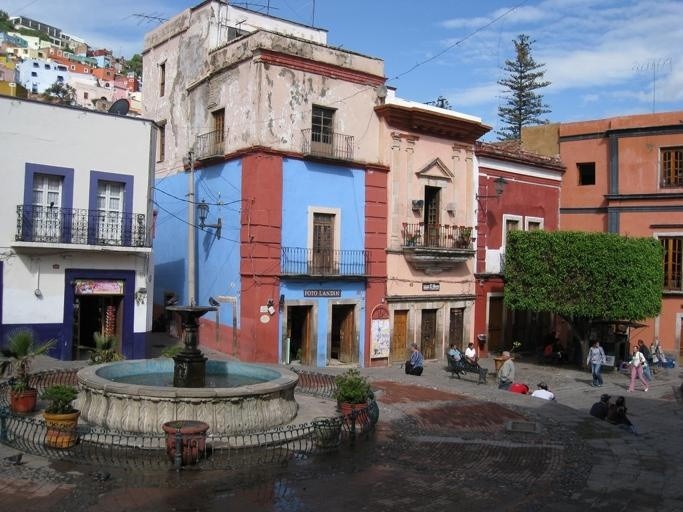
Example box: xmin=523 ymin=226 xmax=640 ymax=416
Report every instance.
xmin=405 ymin=361 xmax=413 ymax=374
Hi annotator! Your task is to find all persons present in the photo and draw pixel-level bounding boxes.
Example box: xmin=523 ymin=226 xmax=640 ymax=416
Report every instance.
xmin=464 ymin=342 xmax=480 ymax=366
xmin=635 ymin=339 xmax=652 ymax=381
xmin=446 ymin=343 xmax=464 ymax=366
xmin=653 ymin=336 xmax=662 ymax=358
xmin=585 ymin=340 xmax=609 ymax=388
xmin=509 ymin=382 xmax=530 ymax=396
xmin=625 ymin=344 xmax=651 ymax=394
xmin=529 ymin=381 xmax=557 ymax=402
xmin=405 ymin=342 xmax=426 ymax=378
xmin=606 ymin=395 xmax=636 ymax=434
xmin=589 ymin=393 xmax=611 ymax=420
xmin=495 ymin=351 xmax=515 ymax=391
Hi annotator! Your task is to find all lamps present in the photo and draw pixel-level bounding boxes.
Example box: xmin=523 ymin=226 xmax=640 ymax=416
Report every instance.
xmin=135 ymin=287 xmax=147 ymax=304
xmin=476 ymin=176 xmax=509 ymax=201
xmin=197 ymin=197 xmax=222 ymax=240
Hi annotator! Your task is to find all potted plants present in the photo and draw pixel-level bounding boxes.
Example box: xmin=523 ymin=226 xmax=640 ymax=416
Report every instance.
xmin=90 ymin=331 xmax=117 ymax=364
xmin=5 ymin=331 xmax=82 ymax=449
xmin=331 ymin=370 xmax=376 ymax=423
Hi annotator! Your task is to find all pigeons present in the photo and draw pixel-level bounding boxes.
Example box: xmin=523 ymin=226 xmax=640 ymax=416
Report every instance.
xmin=87 ymin=471 xmax=112 ymax=483
xmin=2 ymin=453 xmax=25 ymax=465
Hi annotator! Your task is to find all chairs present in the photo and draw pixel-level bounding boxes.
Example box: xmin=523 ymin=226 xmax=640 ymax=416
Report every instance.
xmin=447 ymin=348 xmax=478 ymax=378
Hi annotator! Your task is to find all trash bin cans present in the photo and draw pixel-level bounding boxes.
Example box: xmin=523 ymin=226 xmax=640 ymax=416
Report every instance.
xmin=479 ymin=334 xmax=486 ymax=345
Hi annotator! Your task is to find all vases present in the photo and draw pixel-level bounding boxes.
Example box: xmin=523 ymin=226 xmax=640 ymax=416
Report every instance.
xmin=310 ymin=415 xmax=342 ymax=449
xmin=163 ymin=420 xmax=209 ymax=463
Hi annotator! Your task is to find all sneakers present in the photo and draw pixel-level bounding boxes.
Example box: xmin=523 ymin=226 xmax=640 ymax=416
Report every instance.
xmin=644 ymin=388 xmax=647 ymax=392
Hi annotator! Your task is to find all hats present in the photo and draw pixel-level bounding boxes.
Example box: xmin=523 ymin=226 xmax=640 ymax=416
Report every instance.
xmin=601 ymin=395 xmax=610 ymax=402
xmin=536 ymin=383 xmax=546 ymax=387
xmin=615 ymin=397 xmax=623 ymax=406
